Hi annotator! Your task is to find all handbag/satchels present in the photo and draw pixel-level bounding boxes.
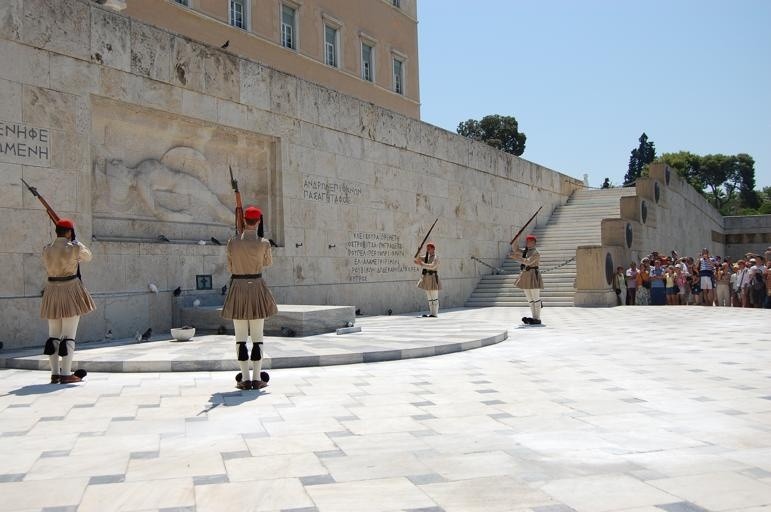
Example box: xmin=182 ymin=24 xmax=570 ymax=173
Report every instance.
xmin=643 ymin=281 xmax=649 ymax=289
xmin=735 ymin=286 xmax=741 ymax=293
xmin=755 ymin=282 xmax=764 ymax=290
xmin=616 ymin=289 xmax=620 ymax=294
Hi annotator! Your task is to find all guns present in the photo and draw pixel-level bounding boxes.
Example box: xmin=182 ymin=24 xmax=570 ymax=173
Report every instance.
xmin=228 ymin=164 xmax=244 ymax=236
xmin=21 ymin=176 xmax=76 ymax=242
xmin=414 ymin=215 xmax=438 ymax=259
xmin=509 ymin=206 xmax=543 ymax=246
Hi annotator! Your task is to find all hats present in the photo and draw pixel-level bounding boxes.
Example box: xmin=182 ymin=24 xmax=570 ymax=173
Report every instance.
xmin=526 ymin=235 xmax=536 ymax=240
xmin=427 ymin=244 xmax=436 ymax=250
xmin=245 ymin=207 xmax=262 ymax=219
xmin=56 ymin=218 xmax=73 ymax=229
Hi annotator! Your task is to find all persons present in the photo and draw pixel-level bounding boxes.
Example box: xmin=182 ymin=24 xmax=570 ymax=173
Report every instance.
xmin=40 ymin=218 xmax=96 ymax=383
xmin=220 ymin=206 xmax=280 ymax=389
xmin=510 ymin=233 xmax=544 ymax=324
xmin=104 ymin=156 xmax=235 ymax=223
xmin=613 ymin=247 xmax=771 ymax=309
xmin=414 ymin=243 xmax=441 ymax=317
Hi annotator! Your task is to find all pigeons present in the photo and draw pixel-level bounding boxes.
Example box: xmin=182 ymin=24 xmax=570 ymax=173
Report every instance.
xmin=158 ymin=235 xmax=221 ymax=246
xmin=388 ymin=308 xmax=392 ymax=316
xmin=92 ymin=324 xmax=227 ymax=343
xmin=345 ymin=321 xmax=353 ymax=327
xmin=279 ymin=326 xmax=296 ymax=337
xmin=220 ymin=40 xmax=230 ymax=48
xmin=269 ymin=239 xmax=277 ymax=247
xmin=92 ymin=235 xmax=96 ymax=241
xmin=149 ymin=283 xmax=227 ymax=308
xmin=355 ymin=309 xmax=360 ymax=315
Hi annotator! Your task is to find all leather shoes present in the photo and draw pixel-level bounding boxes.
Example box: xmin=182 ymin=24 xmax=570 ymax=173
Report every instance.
xmin=237 ymin=380 xmax=252 ymax=390
xmin=252 ymin=379 xmax=268 ymax=389
xmin=522 ymin=317 xmax=541 ymax=324
xmin=51 ymin=374 xmax=61 ymax=383
xmin=60 ymin=374 xmax=81 ymax=384
